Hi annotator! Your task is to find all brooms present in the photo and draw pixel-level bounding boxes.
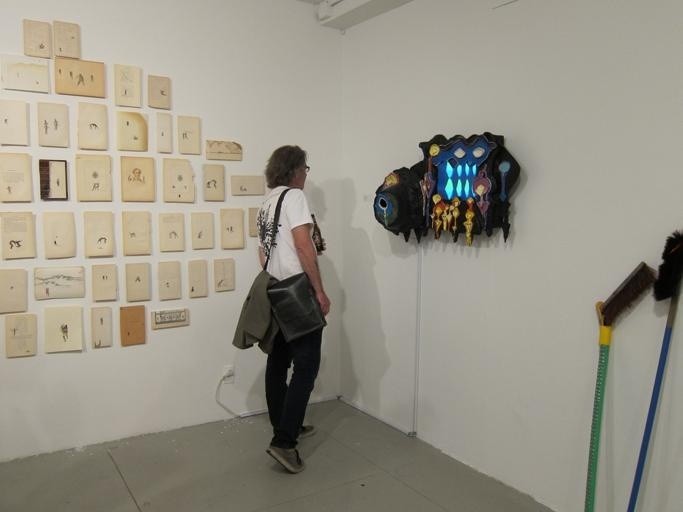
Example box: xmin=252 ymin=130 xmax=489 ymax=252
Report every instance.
xmin=627 ymin=231 xmax=683 ymax=511
xmin=583 ymin=260 xmax=656 ymax=512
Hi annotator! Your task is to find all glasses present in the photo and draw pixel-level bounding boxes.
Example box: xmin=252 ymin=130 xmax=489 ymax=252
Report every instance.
xmin=301 ymin=167 xmax=310 ymax=172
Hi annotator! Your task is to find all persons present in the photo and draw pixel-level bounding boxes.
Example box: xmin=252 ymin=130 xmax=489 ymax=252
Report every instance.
xmin=257 ymin=144 xmax=331 ymax=474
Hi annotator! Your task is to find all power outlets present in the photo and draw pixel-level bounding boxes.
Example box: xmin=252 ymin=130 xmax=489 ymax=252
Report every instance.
xmin=222 ymin=364 xmax=235 ymax=384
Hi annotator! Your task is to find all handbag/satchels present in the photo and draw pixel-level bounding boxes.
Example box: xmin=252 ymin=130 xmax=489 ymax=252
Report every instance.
xmin=267 ymin=272 xmax=328 ymax=343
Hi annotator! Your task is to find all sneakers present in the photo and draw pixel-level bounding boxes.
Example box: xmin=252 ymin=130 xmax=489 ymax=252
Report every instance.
xmin=266 ymin=446 xmax=306 ymax=474
xmin=297 ymin=426 xmax=317 ymax=439
